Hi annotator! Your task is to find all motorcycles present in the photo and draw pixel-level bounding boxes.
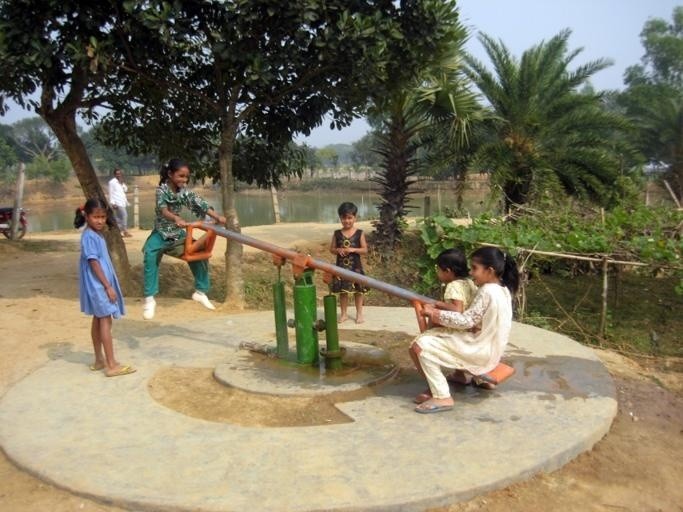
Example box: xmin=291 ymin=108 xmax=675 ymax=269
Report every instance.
xmin=0 ymin=207 xmax=31 ymax=241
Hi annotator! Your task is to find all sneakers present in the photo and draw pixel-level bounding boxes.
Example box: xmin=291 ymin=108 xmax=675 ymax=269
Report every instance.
xmin=192 ymin=292 xmax=216 ymax=311
xmin=143 ymin=300 xmax=156 ymax=320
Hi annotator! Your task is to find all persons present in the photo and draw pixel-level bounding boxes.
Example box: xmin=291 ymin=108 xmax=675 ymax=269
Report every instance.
xmin=329 ymin=202 xmax=368 ymax=323
xmin=410 ymin=246 xmax=520 ymax=414
xmin=73 ymin=198 xmax=137 ymax=377
xmin=407 ymin=248 xmax=482 ymax=405
xmin=108 ymin=169 xmax=134 ymax=238
xmin=142 ymin=158 xmax=227 ymax=320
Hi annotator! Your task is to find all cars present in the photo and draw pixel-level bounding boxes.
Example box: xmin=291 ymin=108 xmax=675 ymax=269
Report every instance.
xmin=626 ymin=156 xmax=671 ymax=175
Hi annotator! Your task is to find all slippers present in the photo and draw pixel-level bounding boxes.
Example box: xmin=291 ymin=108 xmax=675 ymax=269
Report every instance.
xmin=447 ymin=374 xmax=472 ymax=385
xmin=90 ymin=362 xmax=95 ymax=371
xmin=413 ymin=392 xmax=430 ymax=403
xmin=105 ymin=364 xmax=136 ymax=376
xmin=415 ymin=401 xmax=453 ymax=414
